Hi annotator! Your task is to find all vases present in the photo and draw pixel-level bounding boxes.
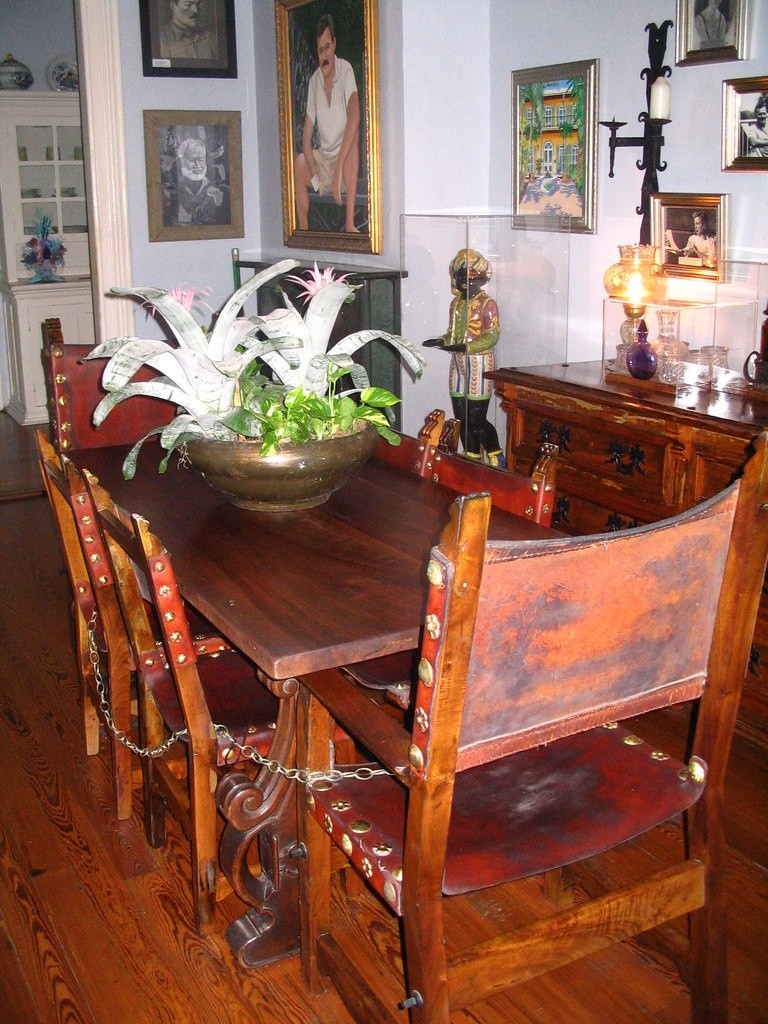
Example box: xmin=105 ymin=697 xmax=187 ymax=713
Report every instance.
xmin=28 ymin=259 xmax=65 ymax=283
xmin=176 ymin=422 xmax=380 ymax=512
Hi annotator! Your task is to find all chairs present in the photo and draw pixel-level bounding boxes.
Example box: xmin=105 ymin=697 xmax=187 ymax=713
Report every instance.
xmin=297 ymin=430 xmax=768 ymax=1024
xmin=431 ymin=417 xmax=559 ymax=527
xmin=373 ymin=409 xmax=445 ymax=479
xmin=31 ymin=426 xmax=237 ymax=821
xmin=41 ymin=318 xmax=188 ymax=451
xmin=81 ymin=469 xmax=366 ymax=938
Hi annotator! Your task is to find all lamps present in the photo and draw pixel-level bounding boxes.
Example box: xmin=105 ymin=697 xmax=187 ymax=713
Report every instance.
xmin=603 ymin=244 xmax=669 ymax=374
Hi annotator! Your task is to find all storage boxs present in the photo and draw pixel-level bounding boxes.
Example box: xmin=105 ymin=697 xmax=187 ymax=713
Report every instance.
xmin=602 ymin=294 xmax=760 ymax=395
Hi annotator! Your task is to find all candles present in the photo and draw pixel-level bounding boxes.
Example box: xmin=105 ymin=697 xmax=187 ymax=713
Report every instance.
xmin=649 ymin=75 xmax=671 ymax=120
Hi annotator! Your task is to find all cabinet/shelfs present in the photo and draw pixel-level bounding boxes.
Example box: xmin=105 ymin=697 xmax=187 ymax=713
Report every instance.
xmin=482 ymin=360 xmax=767 ymax=872
xmin=237 ymin=260 xmax=409 ymax=433
xmin=0 ymin=90 xmax=95 ymax=427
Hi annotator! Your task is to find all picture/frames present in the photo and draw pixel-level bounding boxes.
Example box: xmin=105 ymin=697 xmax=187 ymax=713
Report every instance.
xmin=139 ymin=0 xmax=237 ymax=78
xmin=274 ymin=0 xmax=384 ymax=255
xmin=721 ymin=76 xmax=768 ymax=173
xmin=143 ymin=109 xmax=245 ymax=243
xmin=510 ymin=58 xmax=601 ymax=235
xmin=649 ymin=192 xmax=732 ymax=284
xmin=675 ymin=0 xmax=751 ymax=67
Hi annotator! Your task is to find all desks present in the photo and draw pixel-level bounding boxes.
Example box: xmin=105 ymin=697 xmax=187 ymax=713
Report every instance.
xmin=60 ymin=442 xmax=571 ymax=970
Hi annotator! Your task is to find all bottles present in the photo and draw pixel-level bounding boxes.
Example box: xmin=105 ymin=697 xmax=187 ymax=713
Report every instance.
xmin=650 ymin=310 xmax=690 ymax=375
xmin=659 ymin=350 xmax=677 ymax=385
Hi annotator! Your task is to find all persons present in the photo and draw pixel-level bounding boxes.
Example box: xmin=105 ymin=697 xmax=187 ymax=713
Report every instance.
xmin=666 ymin=211 xmax=716 ymax=270
xmin=745 ymin=104 xmax=768 ymax=158
xmin=422 ymin=248 xmax=506 ymax=470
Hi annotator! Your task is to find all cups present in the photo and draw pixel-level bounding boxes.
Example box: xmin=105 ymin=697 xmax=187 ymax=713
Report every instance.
xmin=700 ymin=346 xmax=715 ymax=367
xmin=74 ymin=146 xmax=83 ymax=160
xmin=46 ymin=147 xmax=61 ymax=161
xmin=17 ymin=146 xmax=26 ymax=161
xmin=713 ymin=345 xmax=728 ymax=368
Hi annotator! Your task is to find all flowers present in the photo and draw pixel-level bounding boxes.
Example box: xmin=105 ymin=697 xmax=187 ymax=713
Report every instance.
xmin=81 ymin=259 xmax=428 ymax=481
xmin=20 ymin=206 xmax=68 ymax=273
